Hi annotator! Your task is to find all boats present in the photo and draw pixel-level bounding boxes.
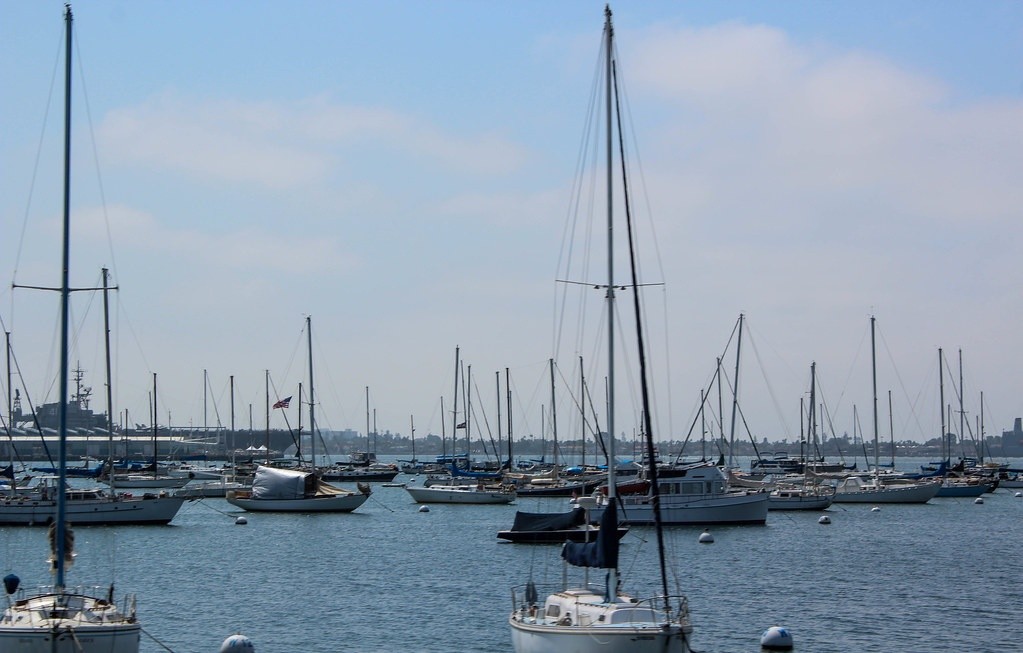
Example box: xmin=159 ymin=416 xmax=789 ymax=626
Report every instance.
xmin=0 ymin=0 xmax=1023 ymax=650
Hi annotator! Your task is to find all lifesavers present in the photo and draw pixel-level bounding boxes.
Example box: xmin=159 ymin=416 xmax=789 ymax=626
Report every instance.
xmin=389 ymin=464 xmax=394 ymax=468
xmin=509 ymin=484 xmax=515 ymax=492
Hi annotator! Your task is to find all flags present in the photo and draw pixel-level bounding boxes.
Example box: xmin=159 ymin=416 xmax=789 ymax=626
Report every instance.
xmin=272 ymin=396 xmax=293 ymax=409
